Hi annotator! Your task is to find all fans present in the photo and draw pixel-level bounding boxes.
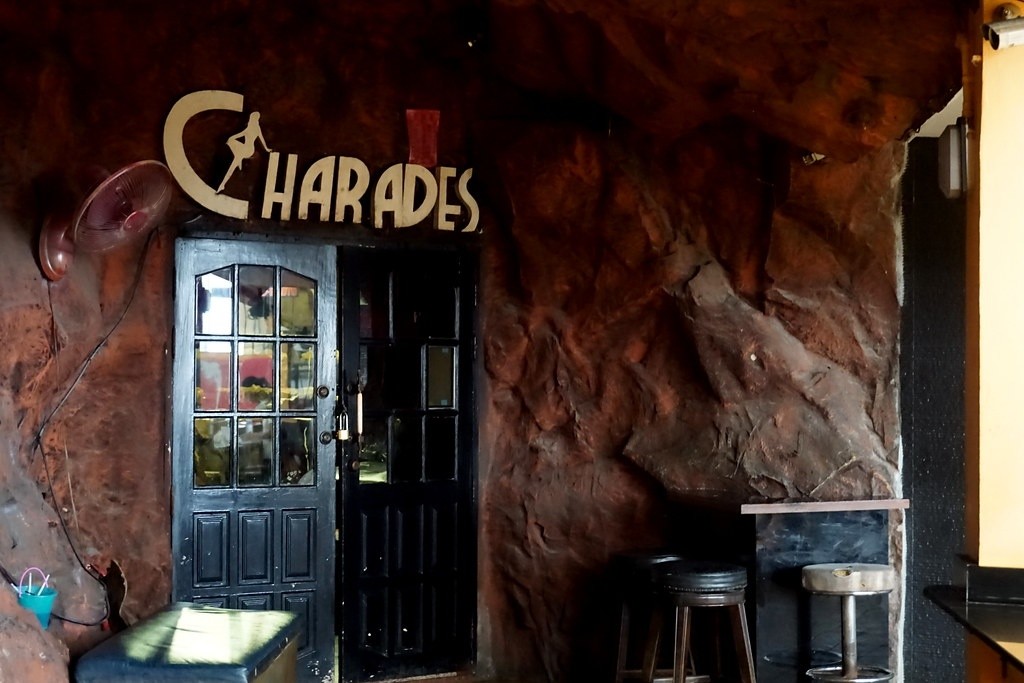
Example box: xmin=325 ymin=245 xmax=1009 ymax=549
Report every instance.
xmin=39 ymin=159 xmax=174 ymax=282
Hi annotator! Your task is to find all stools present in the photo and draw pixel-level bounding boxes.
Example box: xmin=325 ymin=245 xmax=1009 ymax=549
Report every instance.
xmin=803 ymin=563 xmax=896 ymax=683
xmin=764 ymin=549 xmax=852 ymax=683
xmin=607 ymin=548 xmax=756 ymax=683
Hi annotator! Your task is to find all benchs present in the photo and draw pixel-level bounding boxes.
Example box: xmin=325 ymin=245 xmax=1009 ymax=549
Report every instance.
xmin=76 ymin=600 xmax=304 ymax=683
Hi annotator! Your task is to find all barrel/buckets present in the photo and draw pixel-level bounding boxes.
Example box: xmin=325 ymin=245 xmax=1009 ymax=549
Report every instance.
xmin=15 ymin=567 xmax=58 ymax=630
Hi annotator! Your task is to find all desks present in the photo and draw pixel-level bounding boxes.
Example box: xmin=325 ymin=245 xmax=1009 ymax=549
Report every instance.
xmin=923 ymin=585 xmax=1024 ymax=683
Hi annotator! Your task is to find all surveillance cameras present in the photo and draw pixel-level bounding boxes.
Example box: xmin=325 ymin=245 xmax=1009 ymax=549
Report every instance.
xmin=982 ymin=17 xmax=1024 ymax=50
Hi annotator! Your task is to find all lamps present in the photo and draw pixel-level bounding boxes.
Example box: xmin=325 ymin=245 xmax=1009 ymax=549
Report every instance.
xmin=939 ymin=116 xmax=969 ymax=199
